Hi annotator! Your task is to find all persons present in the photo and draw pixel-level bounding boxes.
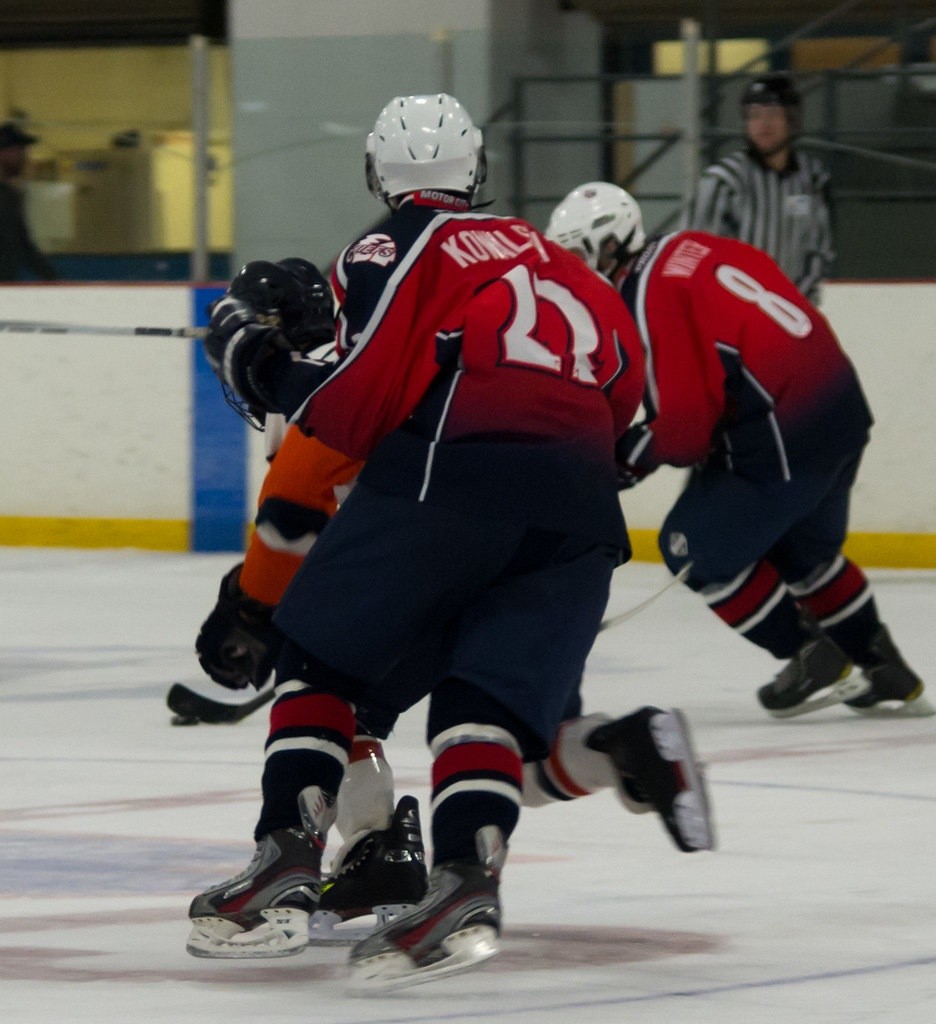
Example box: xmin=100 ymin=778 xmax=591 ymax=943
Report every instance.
xmin=544 ymin=183 xmax=936 ymax=723
xmin=203 ymin=258 xmax=722 ymax=950
xmin=0 ymin=122 xmax=67 ymax=283
xmin=189 ymin=95 xmax=646 ymax=999
xmin=680 ymin=78 xmax=835 ymax=307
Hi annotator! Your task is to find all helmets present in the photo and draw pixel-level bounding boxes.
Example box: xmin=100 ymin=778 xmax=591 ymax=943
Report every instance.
xmin=741 ymin=71 xmax=802 ymax=106
xmin=365 ymin=92 xmax=485 ymax=198
xmin=543 ymin=180 xmax=647 ymax=282
xmin=203 ymin=258 xmax=335 ymax=430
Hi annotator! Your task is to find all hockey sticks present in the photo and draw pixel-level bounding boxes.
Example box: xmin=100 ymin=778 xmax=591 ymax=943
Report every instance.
xmin=0 ymin=318 xmax=216 ymax=346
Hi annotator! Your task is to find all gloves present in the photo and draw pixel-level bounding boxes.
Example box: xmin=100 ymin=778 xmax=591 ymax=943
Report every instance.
xmin=197 ymin=562 xmax=281 ymax=691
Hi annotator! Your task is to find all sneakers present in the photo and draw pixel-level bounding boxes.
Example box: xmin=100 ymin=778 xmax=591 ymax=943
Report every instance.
xmin=759 ymin=622 xmax=936 ymax=718
xmin=187 ymin=701 xmax=714 ymax=985
